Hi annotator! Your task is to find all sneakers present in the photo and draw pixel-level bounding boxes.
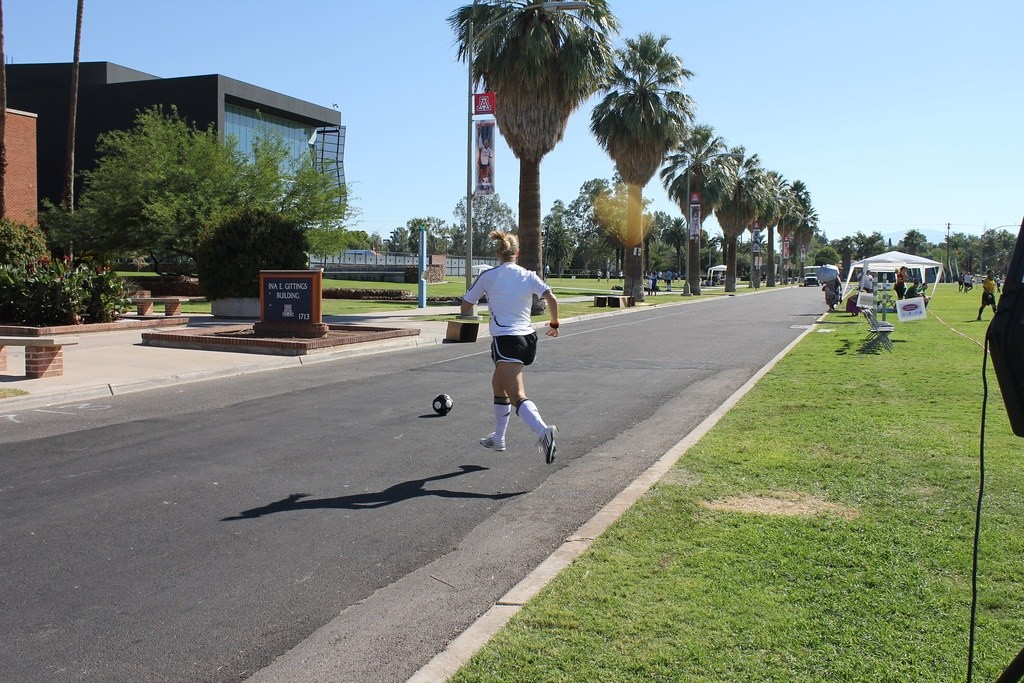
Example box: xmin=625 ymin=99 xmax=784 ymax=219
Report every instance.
xmin=536 ymin=425 xmax=558 ymax=465
xmin=480 ymin=433 xmax=505 ymax=451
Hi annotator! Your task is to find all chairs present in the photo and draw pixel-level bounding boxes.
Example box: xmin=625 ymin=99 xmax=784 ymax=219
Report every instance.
xmin=860 ymin=294 xmax=896 ymax=354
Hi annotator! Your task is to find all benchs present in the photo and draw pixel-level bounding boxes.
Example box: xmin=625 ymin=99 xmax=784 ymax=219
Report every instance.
xmin=0 ymin=337 xmax=78 ymax=378
xmin=131 ymin=298 xmax=190 ymax=315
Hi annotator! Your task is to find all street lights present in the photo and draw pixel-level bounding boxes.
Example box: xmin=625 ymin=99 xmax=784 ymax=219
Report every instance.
xmin=456 ymin=2 xmax=590 ymax=319
xmin=780 ymin=217 xmax=804 ymax=284
xmin=680 ymin=153 xmax=745 ymax=297
xmin=749 ymin=196 xmax=786 ymax=288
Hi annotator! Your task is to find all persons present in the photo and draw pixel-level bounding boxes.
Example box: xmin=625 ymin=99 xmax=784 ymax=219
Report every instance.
xmin=461 ymin=232 xmax=559 ymax=465
xmin=821 ymin=266 xmax=1000 ymax=321
xmin=597 ymin=268 xmax=726 ymax=295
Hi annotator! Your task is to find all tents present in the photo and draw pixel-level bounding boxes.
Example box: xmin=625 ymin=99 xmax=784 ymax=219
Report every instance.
xmin=841 ymin=251 xmax=944 ymax=310
xmin=707 ymin=264 xmax=727 ymax=285
xmin=472 ymin=264 xmax=494 ymax=278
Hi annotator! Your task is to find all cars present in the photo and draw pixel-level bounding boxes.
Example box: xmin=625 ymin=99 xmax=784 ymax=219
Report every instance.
xmin=803 ymin=273 xmax=819 ymax=286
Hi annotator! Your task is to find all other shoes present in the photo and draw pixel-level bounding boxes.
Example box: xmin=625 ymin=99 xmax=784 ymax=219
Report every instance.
xmin=977 ymin=317 xmax=982 ymax=320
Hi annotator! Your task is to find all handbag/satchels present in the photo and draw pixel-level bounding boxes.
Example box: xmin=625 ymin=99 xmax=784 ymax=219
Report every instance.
xmin=894 ymin=285 xmax=897 ymax=290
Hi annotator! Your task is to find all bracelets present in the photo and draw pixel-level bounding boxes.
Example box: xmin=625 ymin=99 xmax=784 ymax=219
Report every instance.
xmin=550 ymin=322 xmax=559 ymax=329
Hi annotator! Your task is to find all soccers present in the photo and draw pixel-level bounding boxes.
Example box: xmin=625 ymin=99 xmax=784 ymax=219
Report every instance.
xmin=432 ymin=393 xmax=454 ymax=415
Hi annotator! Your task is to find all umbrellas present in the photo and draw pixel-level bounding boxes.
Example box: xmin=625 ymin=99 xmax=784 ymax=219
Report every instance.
xmin=815 ymin=264 xmax=839 ymax=282
